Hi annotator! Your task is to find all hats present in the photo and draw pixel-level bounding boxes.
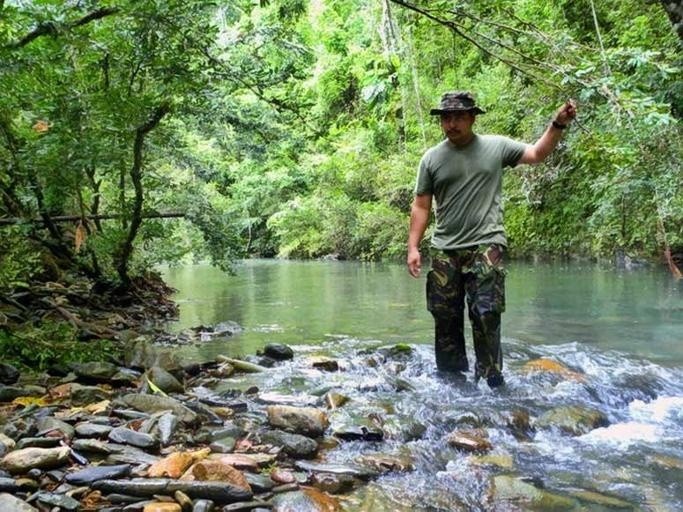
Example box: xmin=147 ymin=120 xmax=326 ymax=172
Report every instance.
xmin=430 ymin=90 xmax=486 ymax=115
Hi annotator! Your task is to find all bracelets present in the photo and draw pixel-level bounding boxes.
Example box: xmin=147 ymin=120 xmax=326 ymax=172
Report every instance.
xmin=552 ymin=121 xmax=569 ymax=130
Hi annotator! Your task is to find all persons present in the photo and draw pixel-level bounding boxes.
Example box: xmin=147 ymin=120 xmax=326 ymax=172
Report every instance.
xmin=406 ymin=92 xmax=577 ymax=390
